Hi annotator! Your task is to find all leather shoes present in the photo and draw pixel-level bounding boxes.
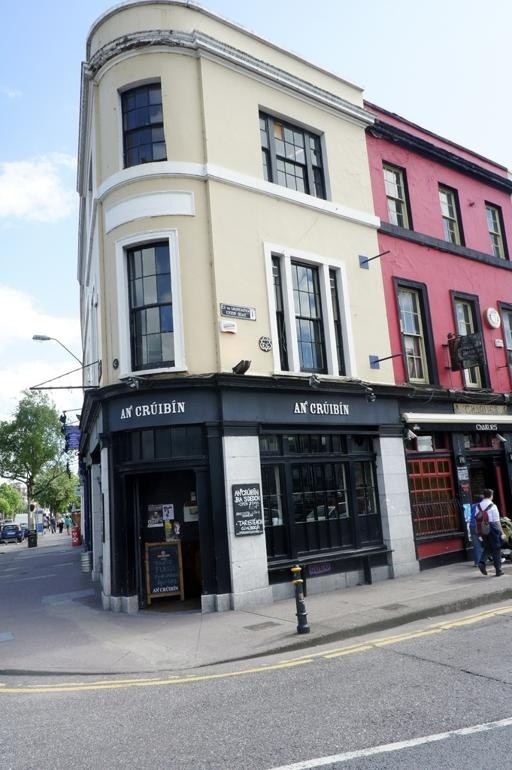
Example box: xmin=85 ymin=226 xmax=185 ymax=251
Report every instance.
xmin=478 ymin=561 xmax=487 ymax=575
xmin=496 ymin=569 xmax=504 ymax=576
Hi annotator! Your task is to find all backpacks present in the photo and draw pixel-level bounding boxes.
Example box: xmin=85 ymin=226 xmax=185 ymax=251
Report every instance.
xmin=474 ymin=503 xmax=495 ymax=537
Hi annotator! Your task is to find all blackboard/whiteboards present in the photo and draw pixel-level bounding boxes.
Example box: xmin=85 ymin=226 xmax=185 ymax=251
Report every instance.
xmin=145 ymin=541 xmax=184 ymax=598
xmin=232 ymin=483 xmax=263 ymax=536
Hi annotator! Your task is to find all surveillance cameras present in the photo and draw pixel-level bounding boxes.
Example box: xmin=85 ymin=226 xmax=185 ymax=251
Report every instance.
xmin=495 ymin=433 xmax=507 ymax=443
xmin=407 ymin=429 xmax=418 ymax=441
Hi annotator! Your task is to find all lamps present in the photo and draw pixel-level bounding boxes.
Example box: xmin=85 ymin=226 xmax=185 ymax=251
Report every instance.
xmin=231 ymin=360 xmax=251 ymax=375
xmin=125 ymin=376 xmax=147 ymax=389
xmin=358 ymin=382 xmax=377 ymax=404
xmin=31 ymin=334 xmax=85 ymax=367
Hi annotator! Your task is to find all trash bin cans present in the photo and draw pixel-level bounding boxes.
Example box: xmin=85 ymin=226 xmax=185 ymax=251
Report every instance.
xmin=28 ymin=530 xmax=37 ymax=548
xmin=72 ymin=526 xmax=80 ymax=546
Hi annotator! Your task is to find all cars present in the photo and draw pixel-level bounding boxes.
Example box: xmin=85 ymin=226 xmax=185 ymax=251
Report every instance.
xmin=304 ymin=501 xmax=358 ymax=521
xmin=0 ymin=519 xmax=28 ymax=544
xmin=263 ymin=507 xmax=281 ymax=526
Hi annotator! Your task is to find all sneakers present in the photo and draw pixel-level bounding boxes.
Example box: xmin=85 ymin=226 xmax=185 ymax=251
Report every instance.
xmin=501 ymin=557 xmax=506 ymax=563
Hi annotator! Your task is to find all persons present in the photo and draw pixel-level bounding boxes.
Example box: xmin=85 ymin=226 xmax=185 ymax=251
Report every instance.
xmin=46 ymin=514 xmax=73 ymax=536
xmin=470 ymin=494 xmax=485 ymax=568
xmin=475 ymin=488 xmax=507 ymax=577
